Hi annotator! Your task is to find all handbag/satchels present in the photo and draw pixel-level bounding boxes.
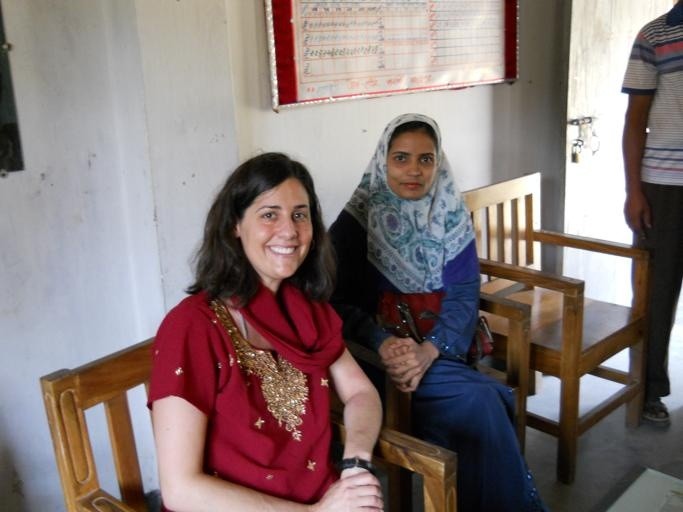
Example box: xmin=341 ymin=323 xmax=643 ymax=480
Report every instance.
xmin=377 ymin=285 xmax=495 ymax=359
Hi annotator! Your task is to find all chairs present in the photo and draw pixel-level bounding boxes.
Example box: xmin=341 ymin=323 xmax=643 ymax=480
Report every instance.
xmin=309 ymin=244 xmax=531 ymax=508
xmin=39 ymin=324 xmax=458 ymax=512
xmin=448 ymin=171 xmax=650 ymax=493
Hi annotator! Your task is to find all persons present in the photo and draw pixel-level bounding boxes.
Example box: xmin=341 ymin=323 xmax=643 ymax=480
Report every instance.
xmin=618 ymin=0 xmax=682 ymax=427
xmin=146 ymin=151 xmax=385 ymax=512
xmin=325 ymin=112 xmax=547 ymax=511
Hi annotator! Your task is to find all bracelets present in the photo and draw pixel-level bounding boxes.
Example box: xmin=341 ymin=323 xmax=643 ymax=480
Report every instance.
xmin=338 ymin=456 xmax=376 ymax=476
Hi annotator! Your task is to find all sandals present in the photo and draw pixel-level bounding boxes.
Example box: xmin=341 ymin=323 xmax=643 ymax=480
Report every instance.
xmin=638 ymin=395 xmax=672 ymax=429
